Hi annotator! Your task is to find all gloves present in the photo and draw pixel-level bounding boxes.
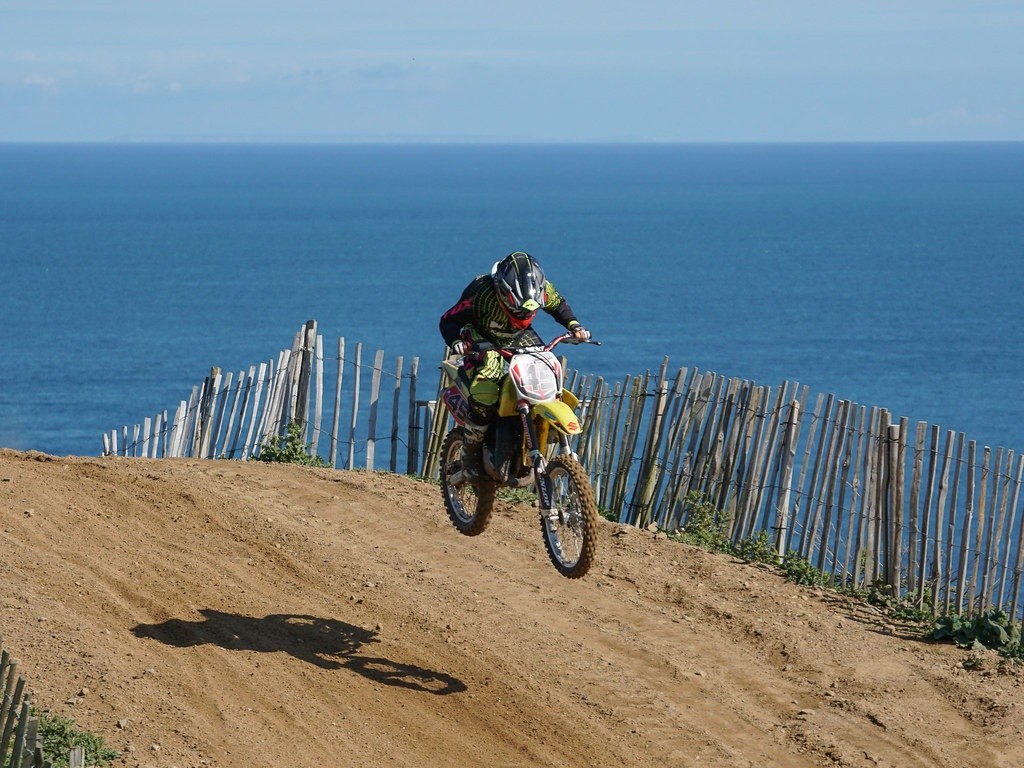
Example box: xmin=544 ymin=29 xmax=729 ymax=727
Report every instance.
xmin=570 ymin=324 xmax=592 ymax=345
xmin=452 ymin=340 xmax=471 ymax=355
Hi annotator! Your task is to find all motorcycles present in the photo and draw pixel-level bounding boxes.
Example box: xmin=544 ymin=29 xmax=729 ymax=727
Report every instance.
xmin=438 ymin=332 xmax=602 ymax=579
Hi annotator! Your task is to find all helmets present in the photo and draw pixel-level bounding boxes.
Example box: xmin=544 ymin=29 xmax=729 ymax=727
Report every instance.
xmin=494 ymin=251 xmax=546 ymax=330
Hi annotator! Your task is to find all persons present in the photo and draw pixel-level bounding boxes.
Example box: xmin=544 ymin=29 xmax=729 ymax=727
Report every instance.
xmin=438 ymin=251 xmax=592 ymax=482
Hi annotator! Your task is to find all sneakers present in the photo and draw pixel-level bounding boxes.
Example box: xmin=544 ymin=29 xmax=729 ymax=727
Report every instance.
xmin=460 ymin=446 xmax=483 ymax=480
